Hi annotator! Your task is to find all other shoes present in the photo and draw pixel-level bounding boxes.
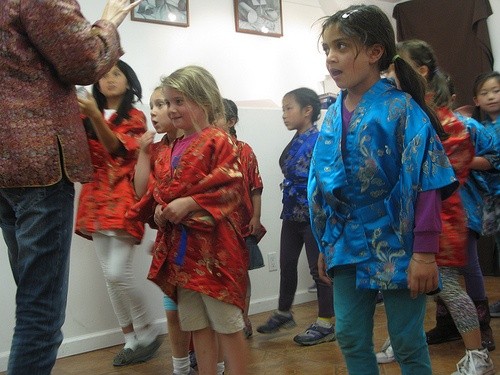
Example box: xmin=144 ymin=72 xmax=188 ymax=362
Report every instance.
xmin=243 ymin=319 xmax=253 ymax=338
xmin=488 ymin=300 xmax=500 ymax=316
xmin=188 ymin=351 xmax=197 ymax=367
xmin=480 ymin=326 xmax=496 ymax=350
xmin=113 ymin=345 xmax=138 ymax=365
xmin=425 ymin=326 xmax=461 ymax=344
xmin=129 ymin=334 xmax=162 ymax=363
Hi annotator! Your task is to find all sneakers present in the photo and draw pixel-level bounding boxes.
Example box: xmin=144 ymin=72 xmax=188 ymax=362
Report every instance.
xmin=256 ymin=312 xmax=297 ymax=333
xmin=449 ymin=348 xmax=494 ymax=375
xmin=375 ymin=337 xmax=395 ymax=363
xmin=294 ymin=324 xmax=336 ymax=346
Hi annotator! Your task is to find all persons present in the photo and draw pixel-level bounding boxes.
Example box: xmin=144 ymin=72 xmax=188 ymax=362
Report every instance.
xmin=307 ymin=4 xmax=457 ymax=375
xmin=375 ymin=39 xmax=500 ymax=375
xmin=0 ymin=0 xmax=143 ymax=375
xmin=75 ymin=59 xmax=166 ymax=366
xmin=257 ymin=87 xmax=337 ymax=345
xmin=130 ymin=66 xmax=268 ymax=375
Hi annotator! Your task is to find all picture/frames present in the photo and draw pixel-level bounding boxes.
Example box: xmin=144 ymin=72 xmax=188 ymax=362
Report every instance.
xmin=130 ymin=0 xmax=190 ymax=28
xmin=233 ymin=0 xmax=283 ymax=38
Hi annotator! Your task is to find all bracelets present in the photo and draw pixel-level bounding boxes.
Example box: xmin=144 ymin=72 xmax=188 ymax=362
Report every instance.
xmin=412 ymin=255 xmax=436 ymax=265
